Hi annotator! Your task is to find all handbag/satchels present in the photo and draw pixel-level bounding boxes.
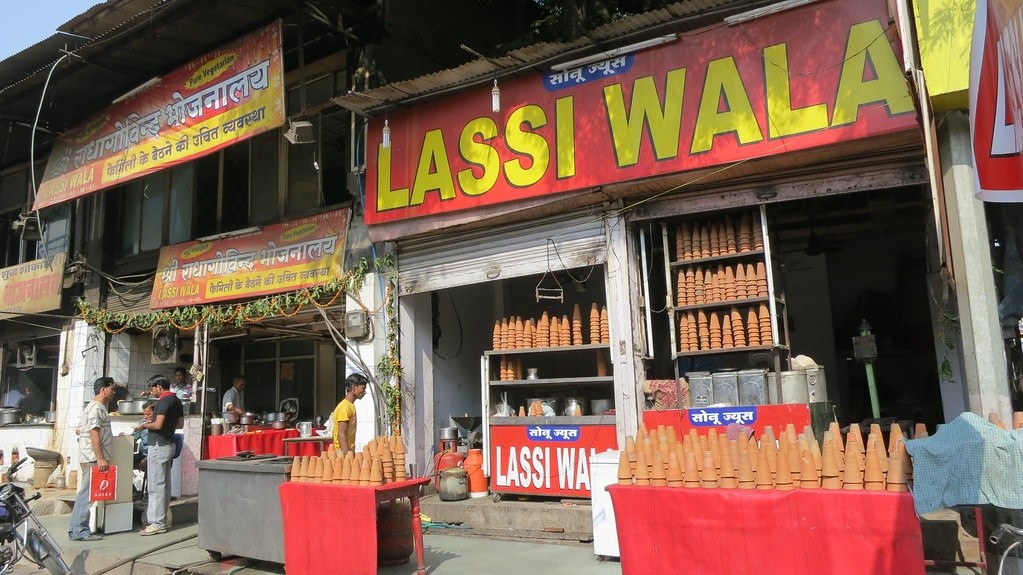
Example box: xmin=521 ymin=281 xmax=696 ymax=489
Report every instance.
xmin=89 ymin=465 xmax=117 ymax=501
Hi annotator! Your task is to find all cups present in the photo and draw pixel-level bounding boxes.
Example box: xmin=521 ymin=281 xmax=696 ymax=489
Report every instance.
xmin=295 ymin=422 xmax=312 ymax=436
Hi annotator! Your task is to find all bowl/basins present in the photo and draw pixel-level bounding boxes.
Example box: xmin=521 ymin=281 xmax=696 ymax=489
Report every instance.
xmin=438 ymin=427 xmax=458 ymax=440
xmin=26 ymin=447 xmax=59 ymax=459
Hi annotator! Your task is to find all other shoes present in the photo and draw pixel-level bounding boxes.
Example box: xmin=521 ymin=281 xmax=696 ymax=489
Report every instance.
xmin=68 ymin=532 xmax=73 ymax=540
xmin=140 ymin=524 xmax=166 ymax=536
xmin=73 ymin=533 xmax=103 ymax=541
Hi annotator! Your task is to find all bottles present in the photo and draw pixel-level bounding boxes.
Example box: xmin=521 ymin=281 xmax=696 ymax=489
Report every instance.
xmin=463 ymin=449 xmax=488 ymax=497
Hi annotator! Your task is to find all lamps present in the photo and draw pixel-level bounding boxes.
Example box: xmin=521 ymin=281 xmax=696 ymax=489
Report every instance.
xmin=12 ymin=213 xmax=45 ymax=241
xmin=112 ymin=76 xmax=162 ymax=104
xmin=551 ymin=33 xmax=678 ymax=72
xmin=211 ymin=328 xmax=250 ymax=340
xmin=724 ymin=0 xmax=819 ymax=26
xmin=284 ymin=121 xmax=317 ymax=144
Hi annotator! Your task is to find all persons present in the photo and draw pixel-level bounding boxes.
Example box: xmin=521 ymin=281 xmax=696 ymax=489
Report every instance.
xmin=68 ymin=377 xmax=115 ymax=541
xmin=140 ymin=367 xmax=192 ymax=405
xmin=134 ymin=374 xmax=182 ymax=536
xmin=119 ymin=400 xmax=157 ymax=502
xmin=333 ymin=373 xmax=368 ymax=454
xmin=222 ymin=375 xmax=247 ymax=416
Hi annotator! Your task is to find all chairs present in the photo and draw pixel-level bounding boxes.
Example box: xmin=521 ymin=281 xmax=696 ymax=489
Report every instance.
xmin=141 ymin=434 xmax=184 ymax=500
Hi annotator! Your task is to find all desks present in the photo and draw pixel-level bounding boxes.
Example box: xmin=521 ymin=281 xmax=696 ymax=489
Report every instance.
xmin=605 ymin=482 xmax=926 ymax=575
xmin=282 ymin=435 xmax=333 ymax=457
xmin=279 ymin=477 xmax=431 ymax=575
xmin=203 ymin=426 xmax=331 ymax=459
xmin=95 ymin=434 xmax=135 ymax=535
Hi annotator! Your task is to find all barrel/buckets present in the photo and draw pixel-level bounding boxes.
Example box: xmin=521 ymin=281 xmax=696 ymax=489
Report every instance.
xmin=526 ymin=396 xmax=613 ymax=416
xmin=436 ymin=466 xmax=468 ymax=501
xmin=34 ymin=458 xmax=58 ymax=488
xmin=685 ymin=364 xmax=828 ymax=406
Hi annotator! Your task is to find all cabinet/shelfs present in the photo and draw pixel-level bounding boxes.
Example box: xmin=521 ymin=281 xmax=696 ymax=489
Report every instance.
xmin=662 ymin=203 xmax=791 ymax=408
xmin=484 ymin=344 xmax=618 ymax=503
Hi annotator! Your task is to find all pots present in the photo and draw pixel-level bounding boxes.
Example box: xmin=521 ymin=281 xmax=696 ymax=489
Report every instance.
xmin=0 ymin=406 xmax=22 ymax=425
xmin=45 ymin=411 xmax=56 ymax=423
xmin=118 ymin=398 xmax=148 ymax=414
xmin=220 ymin=410 xmax=286 ymax=425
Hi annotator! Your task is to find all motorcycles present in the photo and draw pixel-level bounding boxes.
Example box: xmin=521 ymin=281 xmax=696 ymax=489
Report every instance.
xmin=0 ymin=457 xmax=70 ymax=575
